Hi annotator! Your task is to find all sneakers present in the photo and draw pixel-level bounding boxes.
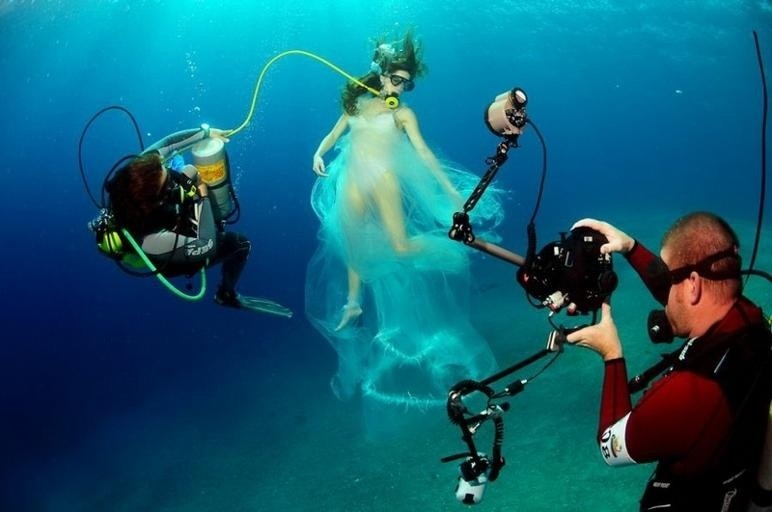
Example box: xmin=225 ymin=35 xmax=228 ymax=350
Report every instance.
xmin=214 ymin=289 xmax=246 ymax=307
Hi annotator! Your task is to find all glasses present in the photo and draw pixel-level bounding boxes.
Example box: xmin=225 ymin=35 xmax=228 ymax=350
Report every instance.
xmin=161 ymin=168 xmax=174 ymax=203
xmin=653 ymin=258 xmax=715 ymax=307
xmin=382 ymin=73 xmax=414 ymax=91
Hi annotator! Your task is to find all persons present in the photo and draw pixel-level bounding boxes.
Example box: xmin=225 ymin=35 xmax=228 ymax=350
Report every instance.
xmin=565 ymin=210 xmax=772 ymax=512
xmin=104 ymin=123 xmax=251 ymax=310
xmin=303 ymin=24 xmax=511 ymax=410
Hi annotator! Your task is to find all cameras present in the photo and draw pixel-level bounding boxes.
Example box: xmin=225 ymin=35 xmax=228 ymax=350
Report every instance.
xmin=517 ymin=225 xmax=618 ymax=315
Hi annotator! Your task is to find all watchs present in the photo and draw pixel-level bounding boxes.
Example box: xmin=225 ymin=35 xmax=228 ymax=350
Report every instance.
xmin=200 ymin=123 xmax=211 ymax=138
